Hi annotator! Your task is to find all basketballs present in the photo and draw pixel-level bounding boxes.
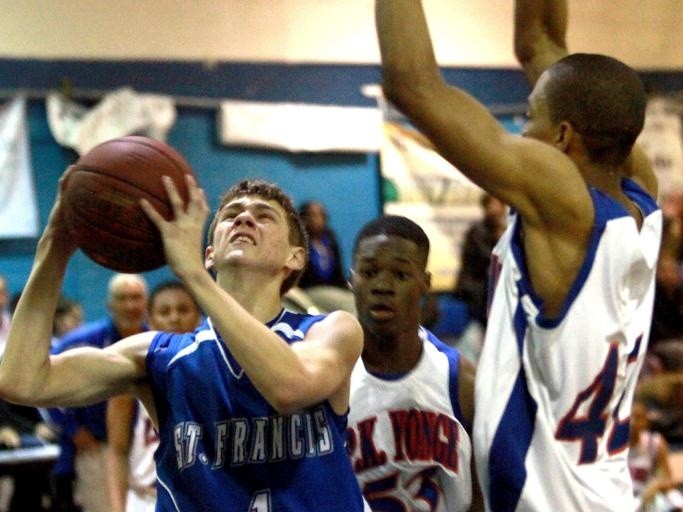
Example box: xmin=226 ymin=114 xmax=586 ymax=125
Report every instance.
xmin=63 ymin=136 xmax=193 ymax=273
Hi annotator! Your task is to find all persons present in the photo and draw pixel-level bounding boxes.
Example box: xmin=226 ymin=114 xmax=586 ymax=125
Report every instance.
xmin=623 ymin=400 xmax=683 ymax=510
xmin=296 ymin=201 xmax=347 ymax=291
xmin=374 ymin=1 xmax=664 ymax=512
xmin=0 ymin=161 xmax=366 ymax=509
xmin=37 ymin=274 xmax=150 ymax=510
xmin=0 ymin=278 xmax=84 ymax=511
xmin=455 ymin=193 xmax=508 ymax=330
xmin=106 ymin=281 xmax=201 ymax=510
